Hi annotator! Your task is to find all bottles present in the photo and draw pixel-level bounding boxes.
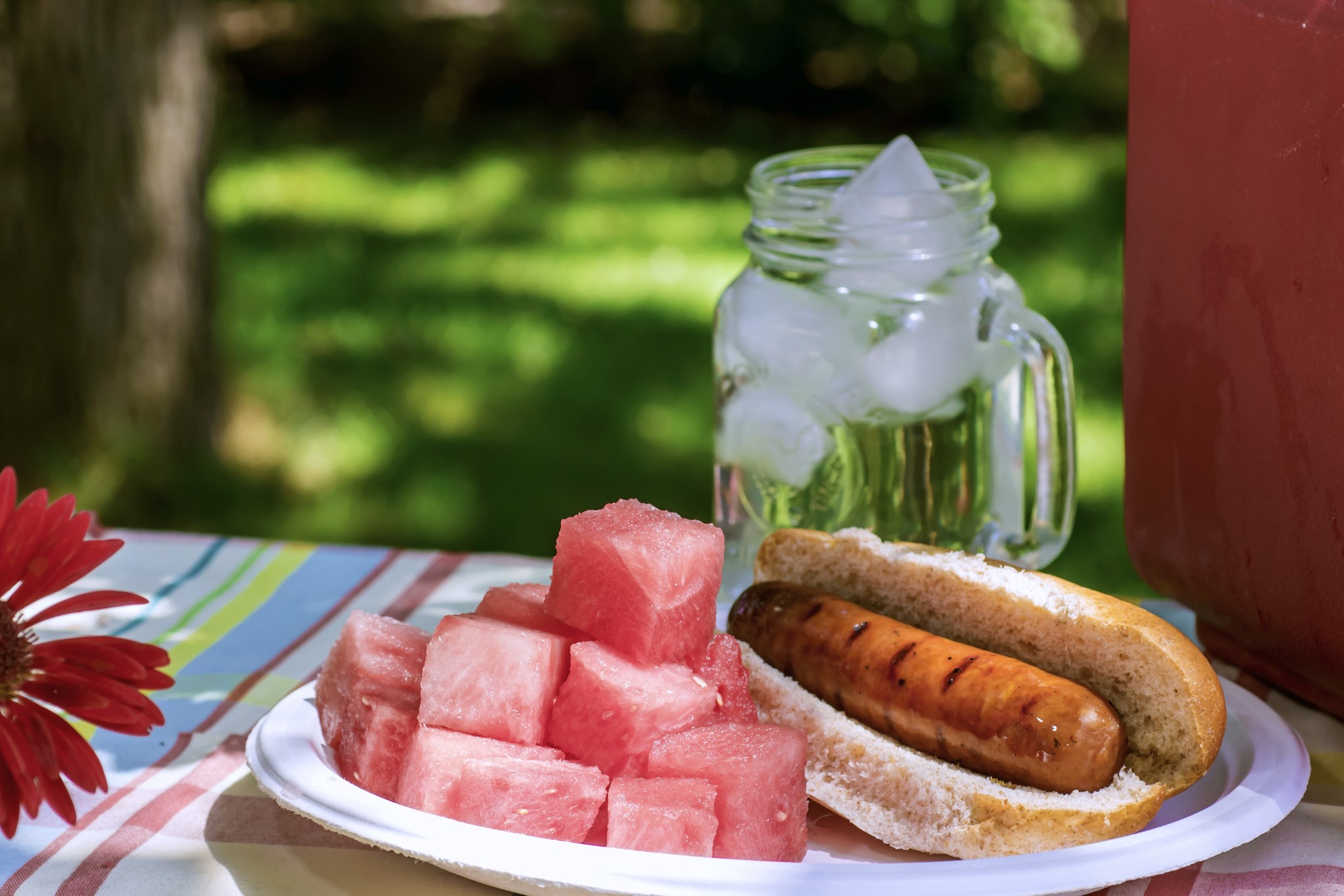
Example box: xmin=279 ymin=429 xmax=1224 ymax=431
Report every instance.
xmin=711 ymin=141 xmax=1077 ymax=632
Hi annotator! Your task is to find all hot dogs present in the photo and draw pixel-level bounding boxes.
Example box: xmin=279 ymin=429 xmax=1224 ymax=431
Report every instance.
xmin=713 ymin=528 xmax=1225 ymax=858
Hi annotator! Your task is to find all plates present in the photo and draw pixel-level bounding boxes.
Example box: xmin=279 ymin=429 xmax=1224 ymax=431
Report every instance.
xmin=244 ymin=672 xmax=1314 ymax=896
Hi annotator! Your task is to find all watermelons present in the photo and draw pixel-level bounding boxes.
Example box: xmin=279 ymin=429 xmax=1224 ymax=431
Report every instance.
xmin=317 ymin=495 xmax=810 ymax=867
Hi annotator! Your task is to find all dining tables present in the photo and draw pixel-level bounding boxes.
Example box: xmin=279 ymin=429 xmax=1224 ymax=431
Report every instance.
xmin=0 ymin=526 xmax=1344 ymax=896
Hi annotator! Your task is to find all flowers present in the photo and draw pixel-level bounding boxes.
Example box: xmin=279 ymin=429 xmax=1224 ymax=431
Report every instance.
xmin=0 ymin=464 xmax=174 ymax=838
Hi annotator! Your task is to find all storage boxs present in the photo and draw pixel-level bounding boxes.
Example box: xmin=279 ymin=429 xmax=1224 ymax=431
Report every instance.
xmin=1123 ymin=0 xmax=1344 ymax=721
